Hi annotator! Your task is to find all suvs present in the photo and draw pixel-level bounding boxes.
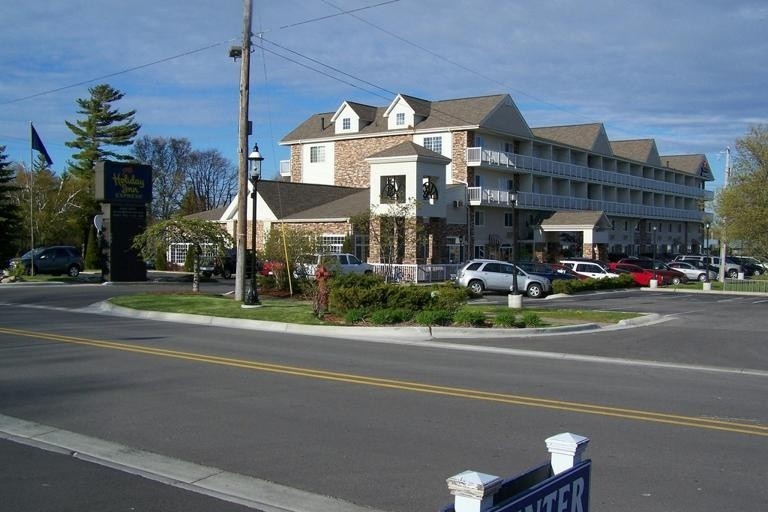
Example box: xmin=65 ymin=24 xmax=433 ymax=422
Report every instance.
xmin=8 ymin=245 xmax=87 ymax=278
xmin=454 ymin=257 xmax=552 ymax=300
xmin=291 ymin=249 xmax=374 ymax=282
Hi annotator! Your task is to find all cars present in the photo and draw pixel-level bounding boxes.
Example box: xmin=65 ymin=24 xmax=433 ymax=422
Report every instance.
xmin=262 ymin=258 xmax=288 ymax=277
xmin=509 ymin=253 xmax=768 ymax=288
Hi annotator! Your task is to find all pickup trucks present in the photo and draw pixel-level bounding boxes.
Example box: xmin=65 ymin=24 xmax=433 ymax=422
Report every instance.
xmin=198 ymin=246 xmax=265 ymax=280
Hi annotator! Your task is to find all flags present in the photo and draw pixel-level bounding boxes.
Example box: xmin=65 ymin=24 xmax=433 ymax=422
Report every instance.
xmin=31 ymin=125 xmax=53 ymax=165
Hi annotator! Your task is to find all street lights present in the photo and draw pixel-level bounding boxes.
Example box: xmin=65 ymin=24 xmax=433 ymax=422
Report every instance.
xmin=648 ymin=225 xmax=659 ymax=289
xmin=242 ymin=140 xmax=267 ymax=309
xmin=702 ymin=222 xmax=713 ymax=291
xmin=505 ymin=185 xmax=523 ymax=309
